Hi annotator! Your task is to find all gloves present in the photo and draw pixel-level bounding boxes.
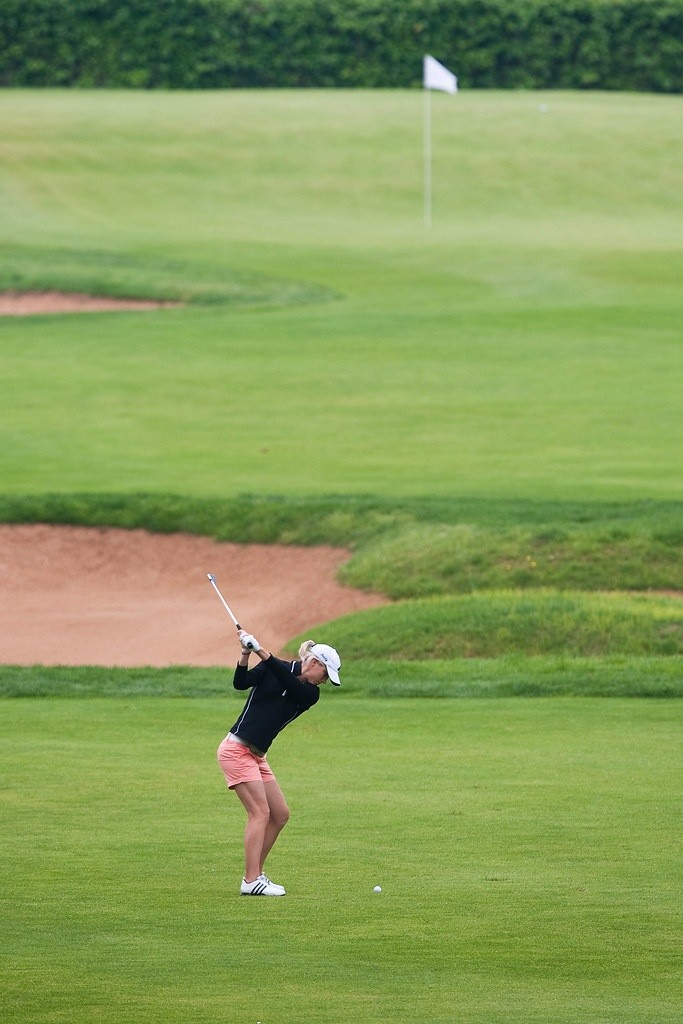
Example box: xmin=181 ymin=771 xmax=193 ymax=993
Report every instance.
xmin=236 ymin=630 xmax=261 ymax=655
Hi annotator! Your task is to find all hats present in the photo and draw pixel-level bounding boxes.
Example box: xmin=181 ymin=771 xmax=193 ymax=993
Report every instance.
xmin=309 ymin=642 xmax=343 ymax=686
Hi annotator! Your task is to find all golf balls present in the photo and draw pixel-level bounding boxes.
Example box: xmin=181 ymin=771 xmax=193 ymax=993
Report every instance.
xmin=373 ymin=885 xmax=382 ymax=892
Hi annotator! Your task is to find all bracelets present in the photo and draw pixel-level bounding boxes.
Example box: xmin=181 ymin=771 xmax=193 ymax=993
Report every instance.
xmin=242 ymin=653 xmax=250 ymax=655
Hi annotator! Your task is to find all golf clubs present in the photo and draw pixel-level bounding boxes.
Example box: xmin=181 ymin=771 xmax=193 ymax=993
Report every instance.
xmin=206 ymin=573 xmax=254 ymax=649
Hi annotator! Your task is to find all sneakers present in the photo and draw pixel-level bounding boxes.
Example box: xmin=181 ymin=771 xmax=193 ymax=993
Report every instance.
xmin=240 ymin=874 xmax=286 ymax=896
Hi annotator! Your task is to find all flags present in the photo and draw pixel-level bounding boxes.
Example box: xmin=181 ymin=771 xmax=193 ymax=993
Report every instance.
xmin=424 ymin=56 xmax=458 ymax=95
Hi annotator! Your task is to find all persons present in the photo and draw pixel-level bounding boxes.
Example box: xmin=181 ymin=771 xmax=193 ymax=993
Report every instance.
xmin=216 ymin=630 xmax=341 ymax=896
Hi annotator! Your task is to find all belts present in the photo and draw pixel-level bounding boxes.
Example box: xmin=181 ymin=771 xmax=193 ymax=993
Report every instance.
xmin=226 ymin=733 xmax=264 ymax=757
